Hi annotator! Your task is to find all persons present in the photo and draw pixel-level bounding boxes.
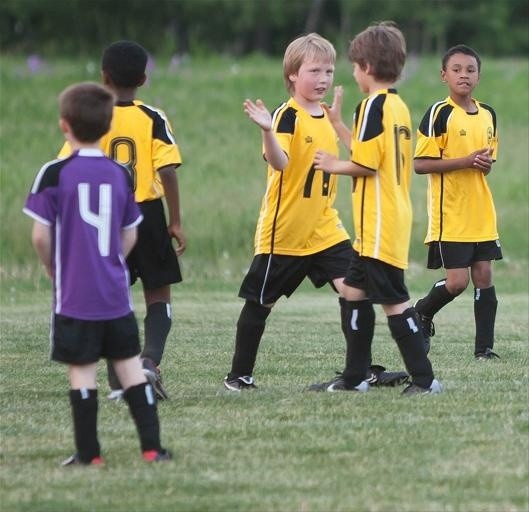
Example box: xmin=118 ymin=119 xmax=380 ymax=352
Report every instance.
xmin=22 ymin=82 xmax=174 ymax=469
xmin=413 ymin=43 xmax=503 ymax=361
xmin=57 ymin=40 xmax=186 ymax=405
xmin=307 ymin=19 xmax=443 ymax=397
xmin=223 ymin=33 xmax=410 ymax=393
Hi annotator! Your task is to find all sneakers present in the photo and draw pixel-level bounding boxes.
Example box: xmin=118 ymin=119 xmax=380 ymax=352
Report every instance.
xmin=402 ymin=379 xmax=440 ymax=395
xmin=222 ymin=374 xmax=256 ymax=391
xmin=475 ymin=347 xmax=500 ymax=360
xmin=310 ymin=365 xmax=409 ymax=392
xmin=413 ymin=297 xmax=435 ymax=353
xmin=144 ymin=449 xmax=172 ymax=461
xmin=141 ymin=356 xmax=167 ymax=402
xmin=61 ymin=455 xmax=102 ymax=464
